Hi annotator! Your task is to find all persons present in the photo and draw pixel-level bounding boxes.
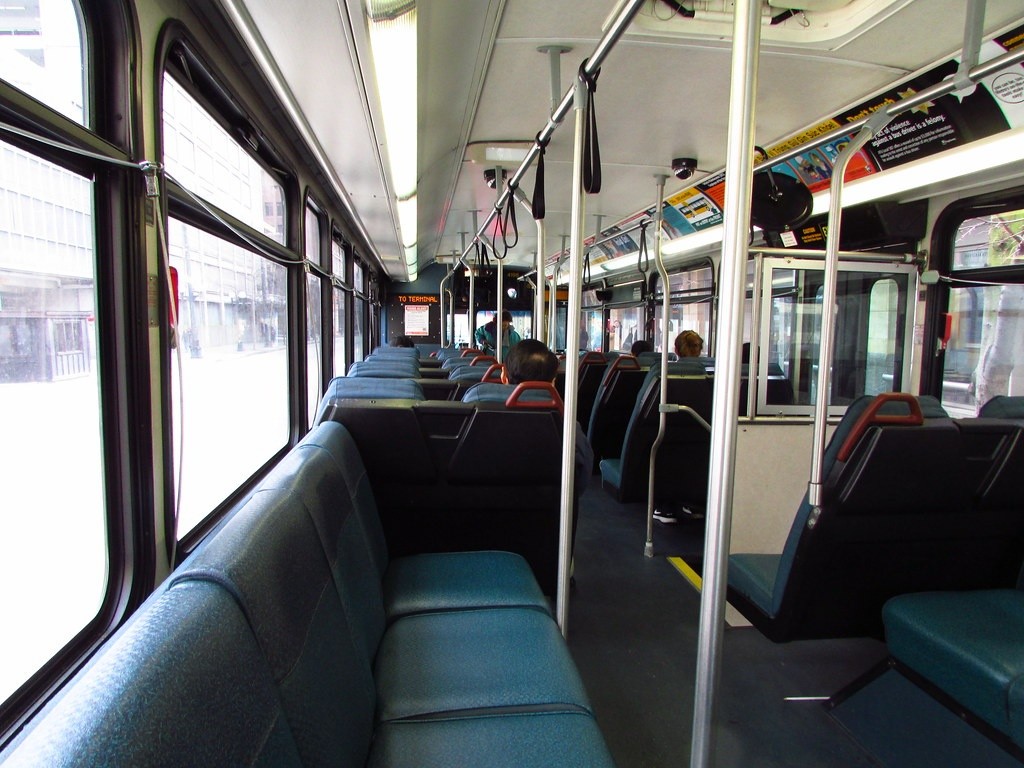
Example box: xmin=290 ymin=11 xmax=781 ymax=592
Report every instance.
xmin=390 ymin=336 xmax=415 ymax=348
xmin=631 ymin=340 xmax=653 ymax=357
xmin=742 ymin=343 xmax=760 ymax=362
xmin=675 ymin=330 xmax=704 ymax=359
xmin=474 ymin=311 xmax=520 ymax=364
xmin=501 ymin=340 xmax=594 ymax=499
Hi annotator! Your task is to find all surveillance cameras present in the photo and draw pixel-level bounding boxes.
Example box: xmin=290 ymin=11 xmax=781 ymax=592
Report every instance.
xmin=672 ymin=158 xmax=698 ymax=180
xmin=484 ymin=169 xmax=507 ymax=190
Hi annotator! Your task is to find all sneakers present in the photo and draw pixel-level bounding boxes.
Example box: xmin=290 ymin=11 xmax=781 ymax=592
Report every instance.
xmin=652 ymin=504 xmax=678 ymax=524
xmin=682 ymin=502 xmax=704 ymax=519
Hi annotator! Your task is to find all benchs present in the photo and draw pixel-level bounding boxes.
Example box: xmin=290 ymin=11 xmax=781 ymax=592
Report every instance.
xmin=1 ymin=342 xmax=1024 ymax=768
